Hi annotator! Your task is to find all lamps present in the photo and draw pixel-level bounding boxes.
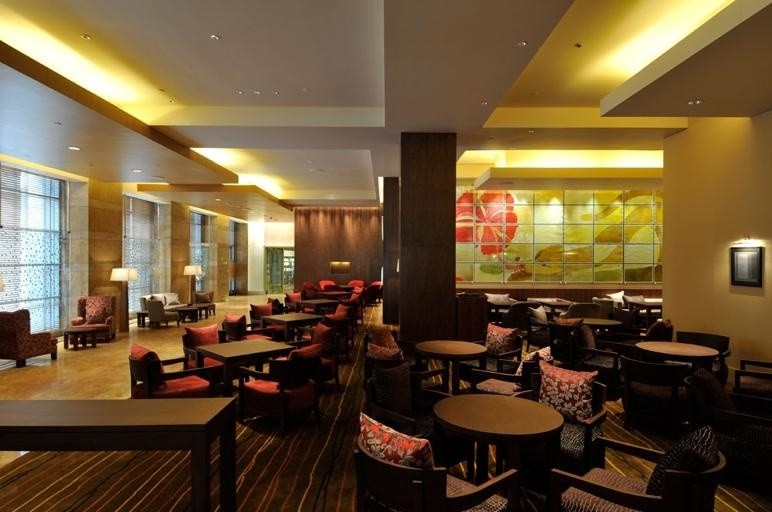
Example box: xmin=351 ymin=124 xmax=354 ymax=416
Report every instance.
xmin=109 ymin=268 xmax=137 ymax=291
xmin=183 ymin=265 xmax=203 ymax=303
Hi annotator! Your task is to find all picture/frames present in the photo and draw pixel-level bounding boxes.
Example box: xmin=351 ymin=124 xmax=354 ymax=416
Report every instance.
xmin=731 ymin=247 xmax=762 ymax=288
